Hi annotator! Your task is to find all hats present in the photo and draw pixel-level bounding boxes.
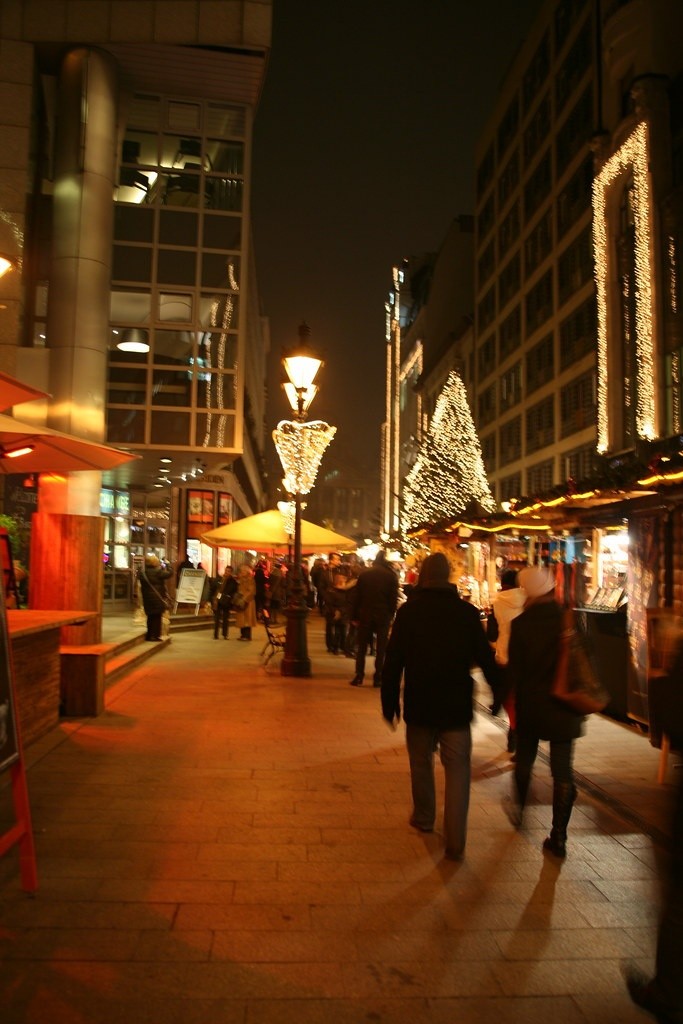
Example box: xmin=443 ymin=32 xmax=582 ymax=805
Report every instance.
xmin=500 ymin=570 xmax=518 ymax=586
xmin=418 ymin=551 xmax=450 ymax=589
xmin=520 ymin=566 xmax=555 ymax=598
xmin=146 ymin=553 xmax=160 ymax=566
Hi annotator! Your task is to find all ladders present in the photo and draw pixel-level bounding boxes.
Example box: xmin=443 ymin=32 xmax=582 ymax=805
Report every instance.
xmin=0 ymin=587 xmax=41 ymax=894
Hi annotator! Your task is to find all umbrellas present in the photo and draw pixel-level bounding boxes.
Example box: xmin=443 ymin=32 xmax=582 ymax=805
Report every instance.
xmin=202 ymin=510 xmax=357 ymax=558
xmin=0 ymin=371 xmax=143 ymax=474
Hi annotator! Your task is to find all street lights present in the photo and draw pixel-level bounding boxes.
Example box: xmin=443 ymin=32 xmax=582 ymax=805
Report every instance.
xmin=271 ymin=344 xmax=339 ymax=678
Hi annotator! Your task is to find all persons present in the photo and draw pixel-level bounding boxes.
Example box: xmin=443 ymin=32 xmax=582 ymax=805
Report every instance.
xmin=621 ymin=639 xmax=683 ymax=1024
xmin=140 ymin=554 xmax=314 ymax=641
xmin=380 ymin=552 xmax=502 ymax=860
xmin=486 ymin=570 xmax=528 ymax=709
xmin=310 ymin=553 xmax=413 ymax=659
xmin=351 ymin=550 xmax=400 ymax=687
xmin=506 ymin=570 xmax=589 ymax=859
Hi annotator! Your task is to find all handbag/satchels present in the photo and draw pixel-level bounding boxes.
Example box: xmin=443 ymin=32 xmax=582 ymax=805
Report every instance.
xmin=553 ymin=629 xmax=612 ymax=709
xmin=232 ymin=592 xmax=248 ymax=611
xmin=160 ymin=592 xmax=176 ymax=609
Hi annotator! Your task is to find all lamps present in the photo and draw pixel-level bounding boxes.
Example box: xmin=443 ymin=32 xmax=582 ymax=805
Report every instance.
xmin=116 ymin=301 xmax=193 ymax=353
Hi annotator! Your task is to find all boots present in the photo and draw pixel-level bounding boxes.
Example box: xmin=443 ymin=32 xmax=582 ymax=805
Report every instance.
xmin=543 ymin=788 xmax=577 ymax=856
xmin=500 ymin=770 xmax=533 ymax=827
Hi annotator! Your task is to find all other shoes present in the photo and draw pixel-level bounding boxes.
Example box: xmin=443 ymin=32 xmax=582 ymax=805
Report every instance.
xmin=224 ymin=635 xmax=230 ymax=640
xmin=237 ymin=637 xmax=252 ymax=641
xmin=146 ymin=635 xmax=161 ymax=641
xmin=507 ymin=726 xmax=517 ymax=753
xmin=409 ymin=813 xmax=435 ymax=832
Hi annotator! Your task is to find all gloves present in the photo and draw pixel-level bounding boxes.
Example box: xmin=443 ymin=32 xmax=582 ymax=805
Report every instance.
xmin=381 ymin=698 xmax=401 ymax=721
xmin=489 ymin=677 xmax=510 ymax=716
xmin=235 ymin=599 xmax=246 ymax=610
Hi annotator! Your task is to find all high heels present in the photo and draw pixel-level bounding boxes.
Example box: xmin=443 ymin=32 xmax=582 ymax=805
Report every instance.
xmin=350 ymin=672 xmax=380 ymax=687
